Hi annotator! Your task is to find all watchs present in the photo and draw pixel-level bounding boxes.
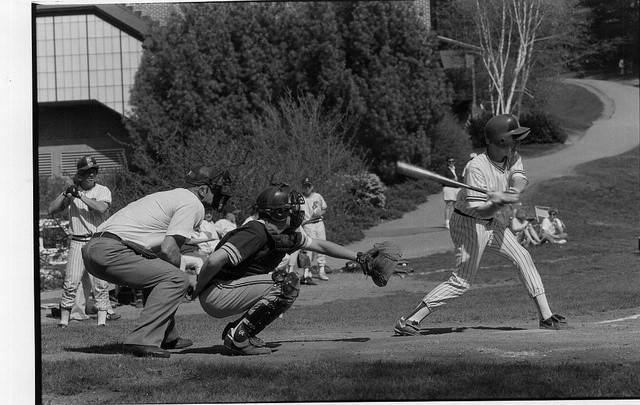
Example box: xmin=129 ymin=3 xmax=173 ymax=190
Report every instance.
xmin=61 ymin=192 xmax=67 ymax=198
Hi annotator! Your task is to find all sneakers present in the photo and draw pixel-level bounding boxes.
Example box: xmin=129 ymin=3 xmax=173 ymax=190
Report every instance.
xmin=222 ymin=322 xmax=237 ymax=340
xmin=300 ymin=276 xmax=318 ymax=285
xmin=540 ymin=314 xmax=567 ymax=330
xmin=318 ymin=270 xmax=328 ymax=280
xmin=222 ymin=327 xmax=271 ymax=355
xmin=394 ymin=317 xmax=422 ymax=337
xmin=106 ymin=313 xmax=121 ymax=320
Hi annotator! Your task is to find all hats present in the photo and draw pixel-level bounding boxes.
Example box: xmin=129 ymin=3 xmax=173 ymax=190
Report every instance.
xmin=470 ymin=152 xmax=478 ymax=160
xmin=548 ymin=209 xmax=558 ymax=213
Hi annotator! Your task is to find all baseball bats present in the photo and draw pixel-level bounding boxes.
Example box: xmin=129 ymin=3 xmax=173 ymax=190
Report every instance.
xmin=396 ymin=159 xmax=488 ymax=195
xmin=67 ymin=193 xmax=72 ymax=199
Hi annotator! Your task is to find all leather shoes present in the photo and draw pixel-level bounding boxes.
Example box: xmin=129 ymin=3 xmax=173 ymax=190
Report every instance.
xmin=124 ymin=343 xmax=170 ymax=358
xmin=161 ymin=337 xmax=193 ymax=348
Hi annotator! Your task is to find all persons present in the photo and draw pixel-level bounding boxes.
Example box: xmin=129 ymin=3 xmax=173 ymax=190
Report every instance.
xmin=442 ymin=155 xmax=461 ymax=230
xmin=48 ymin=155 xmax=112 ymax=328
xmin=195 ymin=207 xmax=222 ymax=262
xmin=393 ymin=114 xmax=569 ymax=337
xmin=539 ymin=208 xmax=568 ymax=244
xmin=295 ymin=177 xmax=329 ymax=285
xmin=461 ymin=153 xmax=478 ymax=177
xmin=509 ymin=210 xmax=545 ymax=246
xmin=185 ymin=185 xmax=400 ymax=356
xmin=215 ymin=205 xmax=240 ymax=239
xmin=81 ymin=165 xmax=234 ymax=358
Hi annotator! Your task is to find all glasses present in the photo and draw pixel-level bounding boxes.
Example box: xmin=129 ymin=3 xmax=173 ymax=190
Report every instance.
xmin=549 ymin=213 xmax=557 ymax=215
xmin=448 ymin=160 xmax=455 ymax=163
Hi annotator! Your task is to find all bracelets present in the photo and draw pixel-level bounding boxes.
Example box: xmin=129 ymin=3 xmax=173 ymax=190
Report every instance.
xmin=356 ymin=251 xmax=362 ymax=263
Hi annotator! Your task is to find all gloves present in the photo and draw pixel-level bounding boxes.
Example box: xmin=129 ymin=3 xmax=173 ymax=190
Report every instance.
xmin=64 ymin=185 xmax=81 ymax=199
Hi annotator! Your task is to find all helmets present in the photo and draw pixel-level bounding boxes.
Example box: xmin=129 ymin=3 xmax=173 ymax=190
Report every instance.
xmin=252 ymin=186 xmax=305 ymax=233
xmin=485 ymin=114 xmax=532 ymax=164
xmin=178 ymin=165 xmax=237 ymax=212
xmin=76 ymin=155 xmax=101 ymax=175
xmin=301 ymin=178 xmax=313 ymax=185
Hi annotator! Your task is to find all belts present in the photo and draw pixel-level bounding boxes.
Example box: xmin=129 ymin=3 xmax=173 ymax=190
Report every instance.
xmin=73 ymin=235 xmax=93 ymax=242
xmin=92 ymin=232 xmax=121 ymax=242
xmin=453 ymin=208 xmax=495 ymax=222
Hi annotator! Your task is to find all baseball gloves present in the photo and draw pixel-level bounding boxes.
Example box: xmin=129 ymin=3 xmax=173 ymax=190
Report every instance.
xmin=355 ymin=240 xmax=403 ymax=287
